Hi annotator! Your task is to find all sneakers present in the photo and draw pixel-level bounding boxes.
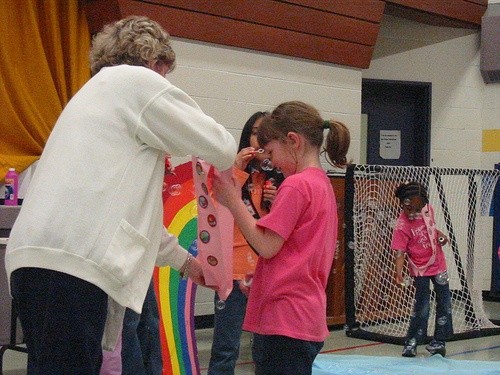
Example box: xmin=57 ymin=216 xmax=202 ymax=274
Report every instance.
xmin=425 ymin=340 xmax=445 ymax=358
xmin=402 ymin=337 xmax=417 ymax=357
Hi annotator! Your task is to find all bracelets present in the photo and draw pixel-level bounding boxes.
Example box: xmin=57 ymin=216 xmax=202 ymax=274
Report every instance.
xmin=180 ymin=252 xmax=194 ymax=282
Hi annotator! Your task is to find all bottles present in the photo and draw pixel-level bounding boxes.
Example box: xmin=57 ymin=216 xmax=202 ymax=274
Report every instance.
xmin=4 ymin=168 xmax=18 ymax=205
xmin=261 ymin=171 xmax=275 ymax=208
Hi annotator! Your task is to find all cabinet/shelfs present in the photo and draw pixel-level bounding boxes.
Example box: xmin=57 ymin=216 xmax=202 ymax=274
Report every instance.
xmin=327 ymin=176 xmax=419 ymax=327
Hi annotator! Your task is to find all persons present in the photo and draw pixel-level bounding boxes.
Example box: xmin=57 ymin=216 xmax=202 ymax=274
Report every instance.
xmin=393 ymin=180 xmax=454 ymax=358
xmin=212 ymin=100 xmax=351 ymax=375
xmin=206 ymin=111 xmax=285 ymax=375
xmin=4 ymin=14 xmax=237 ymax=375
